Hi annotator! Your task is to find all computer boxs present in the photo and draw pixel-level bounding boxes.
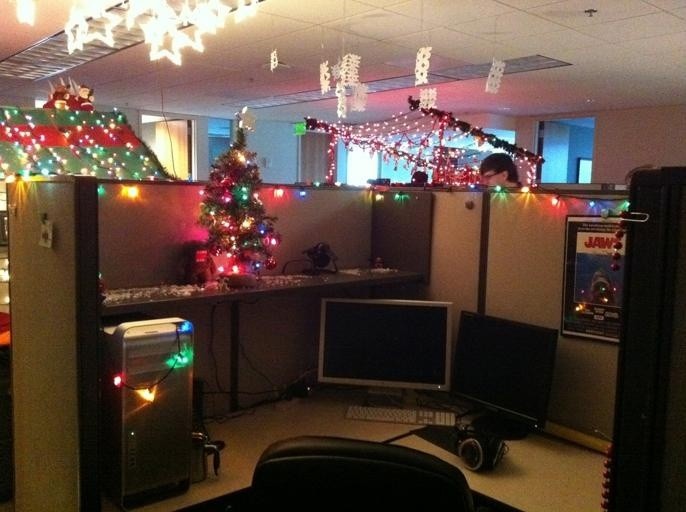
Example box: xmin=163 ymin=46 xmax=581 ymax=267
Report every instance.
xmin=100 ymin=317 xmax=195 ymax=512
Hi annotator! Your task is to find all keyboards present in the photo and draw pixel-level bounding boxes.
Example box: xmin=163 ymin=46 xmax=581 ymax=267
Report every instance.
xmin=345 ymin=405 xmax=456 ymax=427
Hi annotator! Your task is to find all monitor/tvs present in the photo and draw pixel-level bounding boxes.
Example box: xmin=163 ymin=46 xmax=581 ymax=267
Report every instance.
xmin=451 ymin=310 xmax=559 ymax=427
xmin=318 ymin=298 xmax=454 ymax=393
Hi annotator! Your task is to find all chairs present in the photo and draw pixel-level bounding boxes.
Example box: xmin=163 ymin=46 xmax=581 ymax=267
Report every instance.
xmin=250 ymin=435 xmax=476 ymax=511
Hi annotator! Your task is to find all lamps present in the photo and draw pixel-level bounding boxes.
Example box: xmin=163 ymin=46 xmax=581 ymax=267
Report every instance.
xmin=61 ymin=0 xmax=268 ymax=65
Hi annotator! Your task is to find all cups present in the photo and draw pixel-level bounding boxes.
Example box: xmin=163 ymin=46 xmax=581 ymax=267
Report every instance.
xmin=188 ymin=432 xmax=219 ymax=483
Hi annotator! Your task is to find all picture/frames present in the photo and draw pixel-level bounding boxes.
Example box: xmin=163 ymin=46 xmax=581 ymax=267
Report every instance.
xmin=560 ymin=215 xmax=627 ymax=346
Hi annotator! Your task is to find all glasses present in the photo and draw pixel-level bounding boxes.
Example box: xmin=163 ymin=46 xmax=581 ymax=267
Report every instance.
xmin=484 ymin=169 xmax=505 ymax=178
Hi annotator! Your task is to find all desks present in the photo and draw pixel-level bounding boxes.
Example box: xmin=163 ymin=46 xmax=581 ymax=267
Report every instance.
xmin=99 ymin=266 xmax=422 ymax=412
xmin=96 ymin=386 xmax=488 ymax=511
xmin=382 ymin=402 xmax=617 ymax=511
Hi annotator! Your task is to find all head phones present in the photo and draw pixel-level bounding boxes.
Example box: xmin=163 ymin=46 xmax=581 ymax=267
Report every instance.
xmin=455 ymin=430 xmax=507 ymax=470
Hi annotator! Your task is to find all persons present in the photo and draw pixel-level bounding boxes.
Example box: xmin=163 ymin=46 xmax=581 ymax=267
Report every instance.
xmin=480 ymin=153 xmax=522 ymax=186
